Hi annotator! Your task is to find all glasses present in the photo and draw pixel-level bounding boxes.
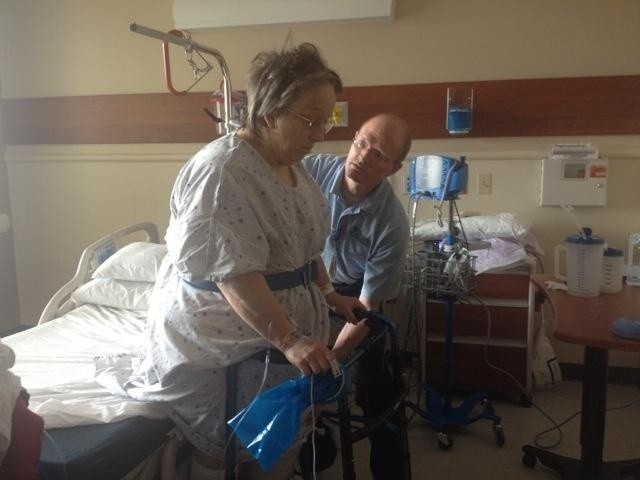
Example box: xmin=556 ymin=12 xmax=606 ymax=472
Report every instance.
xmin=284 ymin=107 xmax=336 ymax=135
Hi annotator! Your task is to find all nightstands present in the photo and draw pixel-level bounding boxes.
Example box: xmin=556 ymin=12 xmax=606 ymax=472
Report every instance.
xmin=417 ymin=252 xmax=537 ymax=408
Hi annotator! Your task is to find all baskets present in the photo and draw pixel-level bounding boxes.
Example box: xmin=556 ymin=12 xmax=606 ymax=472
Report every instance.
xmin=409 ymin=248 xmax=478 ymax=295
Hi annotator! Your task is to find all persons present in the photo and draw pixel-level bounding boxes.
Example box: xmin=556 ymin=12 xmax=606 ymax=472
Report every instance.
xmin=301 ymin=113 xmax=411 ymax=480
xmin=142 ymin=41 xmax=366 ymax=480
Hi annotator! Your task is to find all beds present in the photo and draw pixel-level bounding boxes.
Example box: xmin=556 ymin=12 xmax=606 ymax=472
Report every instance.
xmin=1 ymin=221 xmax=191 ymax=480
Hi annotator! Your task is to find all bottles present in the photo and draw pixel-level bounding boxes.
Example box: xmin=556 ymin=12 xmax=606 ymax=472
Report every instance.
xmin=601 ymin=247 xmax=625 ymax=296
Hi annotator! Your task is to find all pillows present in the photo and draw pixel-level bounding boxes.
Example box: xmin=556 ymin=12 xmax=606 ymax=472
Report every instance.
xmin=69 ymin=241 xmax=167 ymax=311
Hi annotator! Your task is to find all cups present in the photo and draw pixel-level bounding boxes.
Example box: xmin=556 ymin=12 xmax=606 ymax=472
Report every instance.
xmin=553 ymin=227 xmax=607 ymax=299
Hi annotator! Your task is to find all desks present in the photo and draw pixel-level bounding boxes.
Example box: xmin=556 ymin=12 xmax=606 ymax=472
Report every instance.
xmin=522 ymin=273 xmax=640 ymax=480
